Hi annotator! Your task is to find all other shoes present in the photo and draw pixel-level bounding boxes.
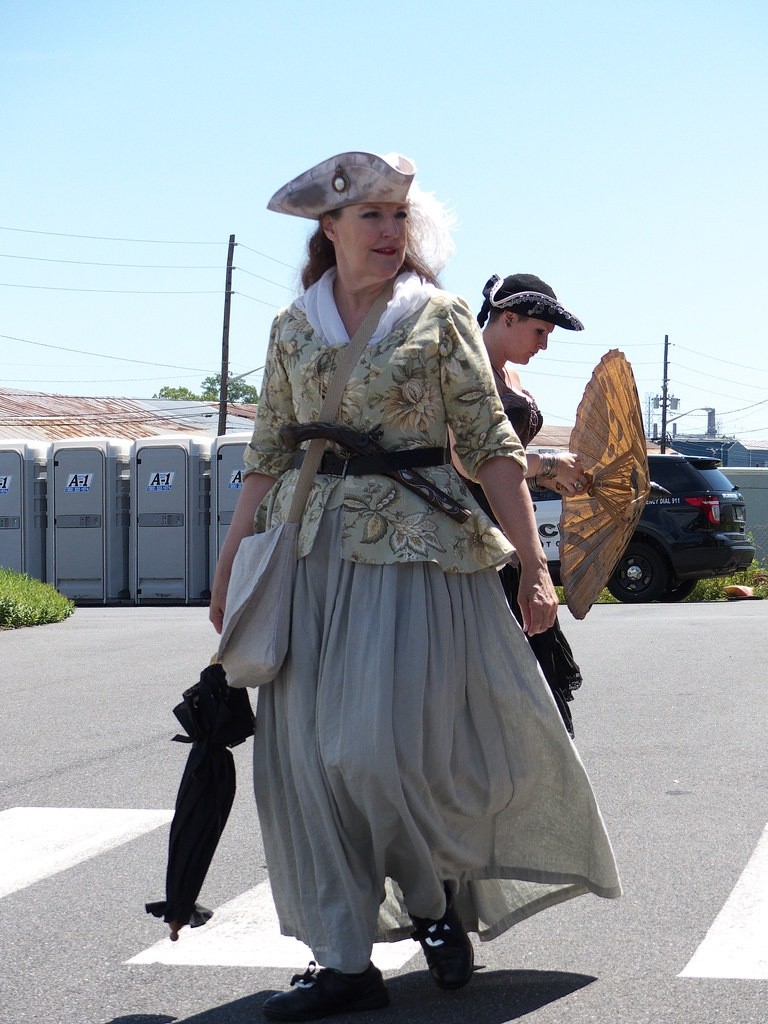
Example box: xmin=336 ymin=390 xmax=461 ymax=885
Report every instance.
xmin=263 ymin=960 xmax=393 ymax=1017
xmin=406 ymin=890 xmax=475 ymax=985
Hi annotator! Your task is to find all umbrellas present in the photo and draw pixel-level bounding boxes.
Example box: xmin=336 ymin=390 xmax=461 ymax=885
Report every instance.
xmin=553 ymin=344 xmax=674 ymax=622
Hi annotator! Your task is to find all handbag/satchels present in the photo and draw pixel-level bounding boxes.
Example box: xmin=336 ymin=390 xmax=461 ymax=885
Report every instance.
xmin=217 ymin=525 xmax=301 ymax=689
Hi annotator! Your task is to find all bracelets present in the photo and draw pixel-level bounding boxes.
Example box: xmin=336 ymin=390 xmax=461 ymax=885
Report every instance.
xmin=525 ymin=475 xmax=548 ymax=492
xmin=534 ymin=451 xmax=560 ymax=479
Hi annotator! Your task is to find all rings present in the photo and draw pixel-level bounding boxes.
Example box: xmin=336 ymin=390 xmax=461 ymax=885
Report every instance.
xmin=572 ymin=479 xmax=584 ymax=490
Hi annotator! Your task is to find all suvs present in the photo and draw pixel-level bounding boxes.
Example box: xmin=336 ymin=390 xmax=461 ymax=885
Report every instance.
xmin=521 ymin=454 xmax=755 ymax=603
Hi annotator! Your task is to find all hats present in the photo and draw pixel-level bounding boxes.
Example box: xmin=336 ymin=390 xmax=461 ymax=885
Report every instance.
xmin=269 ymin=149 xmax=417 ymax=219
xmin=481 ymin=274 xmax=585 ymax=331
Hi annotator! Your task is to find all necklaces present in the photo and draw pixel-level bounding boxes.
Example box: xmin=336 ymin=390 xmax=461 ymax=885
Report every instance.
xmin=490 ymin=360 xmax=512 ymax=389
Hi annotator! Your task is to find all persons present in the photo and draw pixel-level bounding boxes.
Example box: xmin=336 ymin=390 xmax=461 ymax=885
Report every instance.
xmin=441 ymin=271 xmax=593 ymax=743
xmin=204 ymin=143 xmax=628 ymax=1015
